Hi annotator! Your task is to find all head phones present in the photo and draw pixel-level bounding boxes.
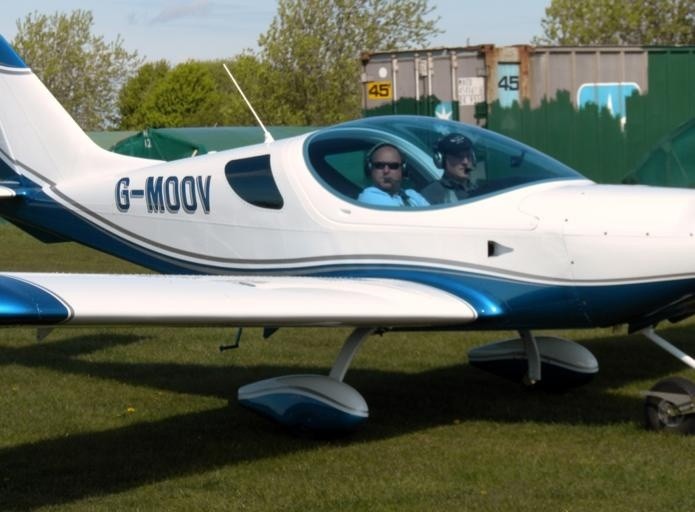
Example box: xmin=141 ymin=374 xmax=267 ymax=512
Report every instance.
xmin=364 ymin=143 xmax=413 ymax=177
xmin=433 ymin=133 xmax=480 ymax=169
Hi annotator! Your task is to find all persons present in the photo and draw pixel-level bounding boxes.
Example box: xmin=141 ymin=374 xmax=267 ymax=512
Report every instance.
xmin=355 ymin=140 xmax=431 ymax=208
xmin=420 ymin=132 xmax=478 ymax=206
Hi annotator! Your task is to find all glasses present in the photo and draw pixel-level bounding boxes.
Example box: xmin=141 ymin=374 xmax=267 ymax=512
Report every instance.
xmin=375 ymin=161 xmax=404 ymax=169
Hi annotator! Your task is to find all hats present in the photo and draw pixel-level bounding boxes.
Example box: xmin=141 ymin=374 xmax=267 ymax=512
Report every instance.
xmin=438 ymin=134 xmax=471 ymax=153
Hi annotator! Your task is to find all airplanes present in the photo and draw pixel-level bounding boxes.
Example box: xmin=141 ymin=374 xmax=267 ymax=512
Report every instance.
xmin=0 ymin=31 xmax=695 ymax=440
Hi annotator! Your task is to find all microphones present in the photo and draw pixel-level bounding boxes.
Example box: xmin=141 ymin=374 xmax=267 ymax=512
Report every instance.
xmin=464 ymin=165 xmax=478 ymax=173
xmin=385 ymin=176 xmax=411 ymax=184
